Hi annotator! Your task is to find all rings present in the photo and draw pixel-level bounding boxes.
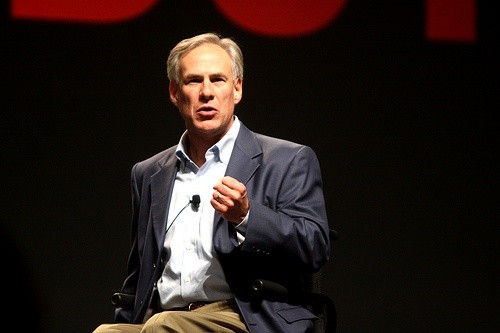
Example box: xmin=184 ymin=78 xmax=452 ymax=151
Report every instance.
xmin=216 ymin=193 xmax=222 ymax=202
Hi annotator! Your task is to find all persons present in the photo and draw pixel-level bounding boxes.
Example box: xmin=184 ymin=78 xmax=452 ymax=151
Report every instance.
xmin=90 ymin=32 xmax=331 ymax=333
